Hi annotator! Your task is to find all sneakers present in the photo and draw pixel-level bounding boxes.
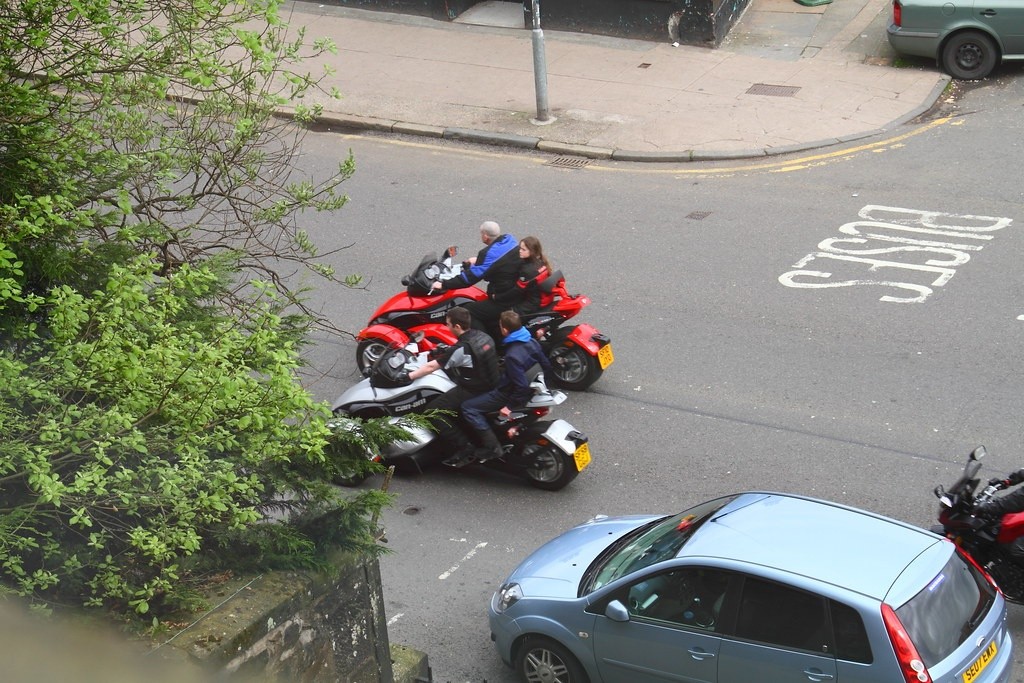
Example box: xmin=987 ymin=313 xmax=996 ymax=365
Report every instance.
xmin=441 ymin=443 xmax=473 ymax=467
xmin=476 ymin=446 xmax=505 ymax=464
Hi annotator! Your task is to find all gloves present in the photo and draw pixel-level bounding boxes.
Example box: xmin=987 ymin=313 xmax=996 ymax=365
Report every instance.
xmin=1007 ymin=471 xmax=1023 ymax=483
xmin=394 ymin=371 xmax=413 ymax=384
xmin=437 ymin=343 xmax=448 ymax=351
xmin=978 ymin=503 xmax=996 ymax=520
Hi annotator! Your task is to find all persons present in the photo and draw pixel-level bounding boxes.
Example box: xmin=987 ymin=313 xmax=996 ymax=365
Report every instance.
xmin=461 ymin=311 xmax=552 ymax=460
xmin=976 ymin=468 xmax=1024 ymax=599
xmin=431 ymin=221 xmax=554 ymax=365
xmin=395 ymin=306 xmax=501 ymax=463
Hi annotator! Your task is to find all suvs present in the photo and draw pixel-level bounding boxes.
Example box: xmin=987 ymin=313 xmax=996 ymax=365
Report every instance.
xmin=487 ymin=488 xmax=1012 ymax=683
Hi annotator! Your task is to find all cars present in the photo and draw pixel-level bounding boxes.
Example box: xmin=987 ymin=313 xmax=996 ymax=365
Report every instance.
xmin=887 ymin=0 xmax=1024 ymax=81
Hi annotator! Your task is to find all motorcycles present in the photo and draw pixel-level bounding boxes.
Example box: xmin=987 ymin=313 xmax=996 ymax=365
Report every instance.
xmin=928 ymin=446 xmax=1024 ymax=604
xmin=357 ymin=245 xmax=615 ymax=391
xmin=323 ymin=328 xmax=593 ymax=491
